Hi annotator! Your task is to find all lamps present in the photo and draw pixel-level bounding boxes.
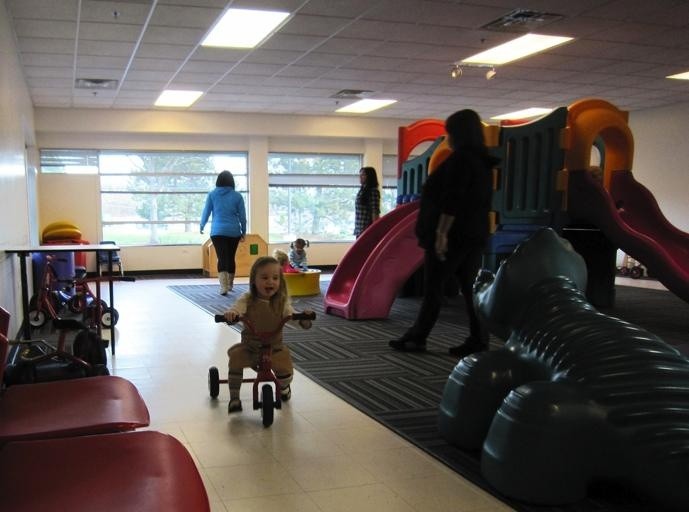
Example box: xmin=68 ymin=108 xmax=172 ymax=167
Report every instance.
xmin=451 ymin=65 xmax=496 ymax=81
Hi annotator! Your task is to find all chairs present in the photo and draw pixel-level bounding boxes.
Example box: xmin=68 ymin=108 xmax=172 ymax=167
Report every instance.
xmin=0 ymin=307 xmax=150 ymax=438
xmin=0 ymin=431 xmax=210 ymax=512
xmin=98 ymin=241 xmax=124 ymax=277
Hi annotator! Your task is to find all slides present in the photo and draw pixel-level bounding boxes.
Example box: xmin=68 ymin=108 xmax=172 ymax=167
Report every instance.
xmin=601 ymin=171 xmax=689 ymax=302
xmin=324 ymin=200 xmax=425 ymax=320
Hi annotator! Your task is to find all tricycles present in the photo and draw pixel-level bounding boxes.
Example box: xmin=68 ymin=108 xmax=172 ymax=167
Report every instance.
xmin=24 ymin=252 xmax=119 ymax=330
xmin=206 ymin=309 xmax=317 ymax=431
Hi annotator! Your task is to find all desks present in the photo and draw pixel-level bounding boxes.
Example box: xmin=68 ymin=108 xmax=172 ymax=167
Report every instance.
xmin=5 ymin=244 xmax=121 ymax=356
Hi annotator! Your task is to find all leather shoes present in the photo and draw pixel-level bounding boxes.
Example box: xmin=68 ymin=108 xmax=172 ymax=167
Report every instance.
xmin=281 ymin=384 xmax=291 ymax=401
xmin=228 ymin=398 xmax=242 ymax=412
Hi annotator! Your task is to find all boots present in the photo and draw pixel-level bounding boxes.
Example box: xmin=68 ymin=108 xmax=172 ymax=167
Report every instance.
xmin=218 ymin=271 xmax=234 ymax=295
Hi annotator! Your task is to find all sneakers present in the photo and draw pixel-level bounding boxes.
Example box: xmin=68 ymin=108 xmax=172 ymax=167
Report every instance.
xmin=449 ymin=337 xmax=489 ymax=356
xmin=389 ymin=333 xmax=425 ymax=351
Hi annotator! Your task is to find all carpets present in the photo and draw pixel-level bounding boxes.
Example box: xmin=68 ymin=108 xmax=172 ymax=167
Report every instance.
xmin=166 ymin=279 xmax=689 ymax=511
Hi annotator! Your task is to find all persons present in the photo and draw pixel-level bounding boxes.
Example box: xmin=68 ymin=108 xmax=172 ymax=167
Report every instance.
xmin=224 ymin=255 xmax=313 ymax=415
xmin=387 ymin=110 xmax=506 ymax=358
xmin=288 ymin=238 xmax=309 ymax=271
xmin=353 ymin=166 xmax=381 ymax=239
xmin=274 ymin=249 xmax=303 ymax=273
xmin=199 ymin=170 xmax=247 ymax=295
